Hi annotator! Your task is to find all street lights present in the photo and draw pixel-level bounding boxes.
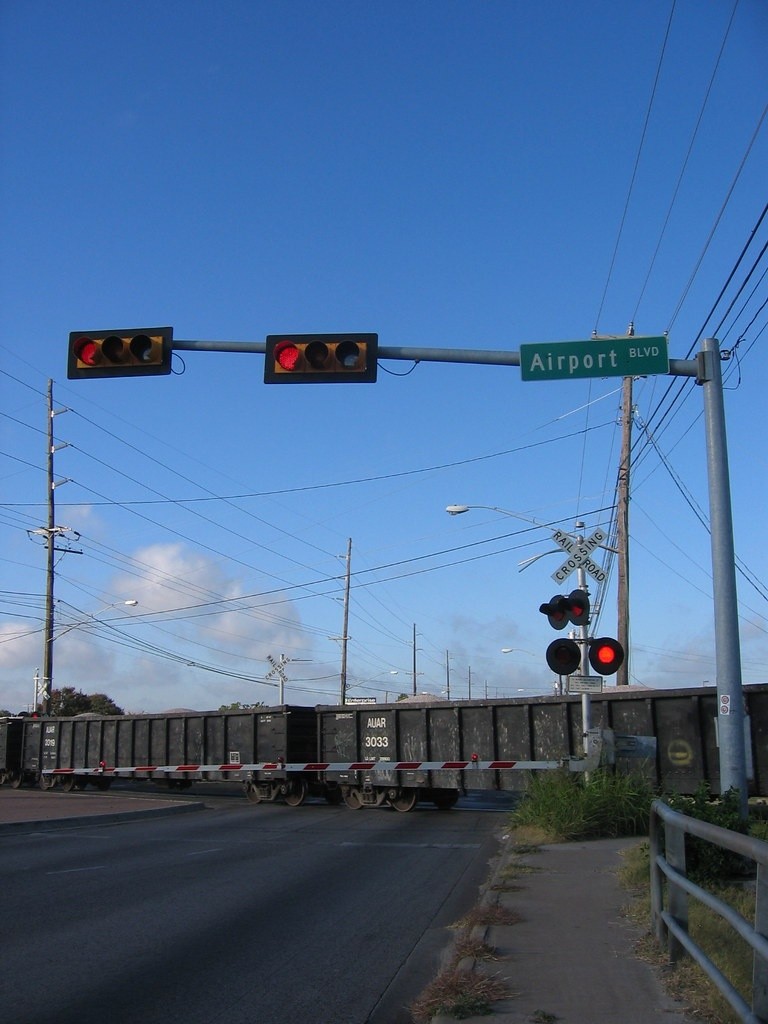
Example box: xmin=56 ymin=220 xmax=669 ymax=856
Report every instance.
xmin=341 ymin=670 xmax=399 ymax=706
xmin=501 ymin=647 xmax=563 ymax=695
xmin=42 ymin=600 xmax=139 ymax=717
xmin=445 ymin=502 xmax=630 ymax=688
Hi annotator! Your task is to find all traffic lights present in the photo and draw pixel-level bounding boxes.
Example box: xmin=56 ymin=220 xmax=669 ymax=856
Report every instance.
xmin=66 ymin=326 xmax=174 ymax=381
xmin=30 ymin=711 xmax=40 ymax=717
xmin=18 ymin=711 xmax=29 ymax=717
xmin=590 ymin=637 xmax=625 ymax=675
xmin=264 ymin=333 xmax=379 ymax=384
xmin=558 ymin=589 xmax=590 ymax=626
xmin=546 ymin=638 xmax=582 ymax=675
xmin=539 ymin=595 xmax=570 ymax=631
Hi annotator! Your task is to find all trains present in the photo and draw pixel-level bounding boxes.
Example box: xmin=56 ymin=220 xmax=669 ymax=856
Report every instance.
xmin=0 ymin=682 xmax=768 ymax=813
